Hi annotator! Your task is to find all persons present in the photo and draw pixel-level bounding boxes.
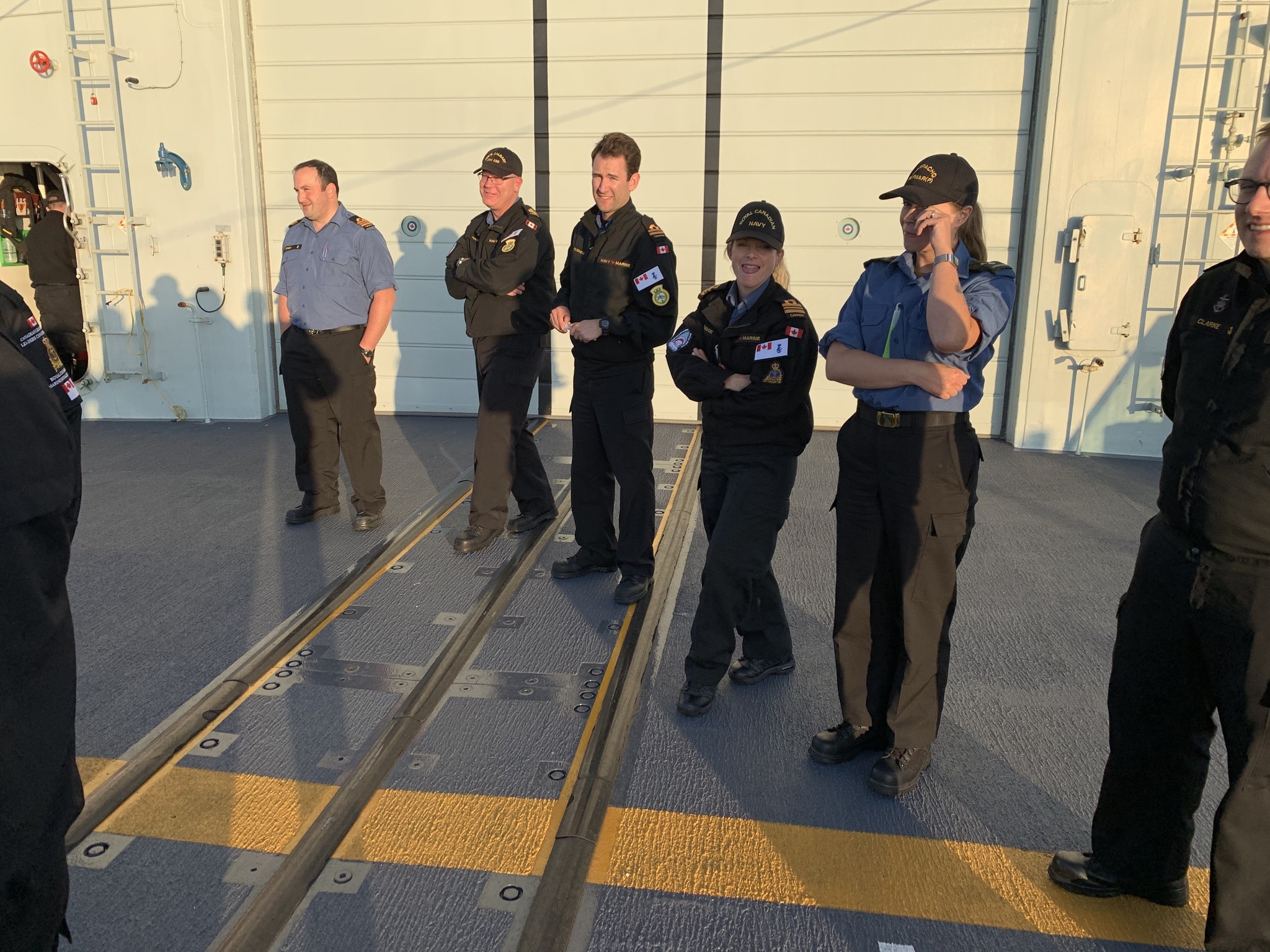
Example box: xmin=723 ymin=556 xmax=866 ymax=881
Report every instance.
xmin=1047 ymin=123 xmax=1270 ymax=952
xmin=550 ymin=132 xmax=678 ymax=603
xmin=0 ymin=279 xmax=86 ymax=952
xmin=445 ymin=147 xmax=559 ymax=552
xmin=666 ymin=200 xmax=819 ymax=715
xmin=26 ymin=191 xmax=88 ymax=383
xmin=808 ymin=153 xmax=1016 ymax=794
xmin=273 ymin=160 xmax=398 ymax=530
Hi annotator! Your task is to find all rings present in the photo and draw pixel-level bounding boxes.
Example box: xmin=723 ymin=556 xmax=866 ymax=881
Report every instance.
xmin=930 ymin=211 xmax=935 ymax=218
xmin=552 ymin=323 xmax=555 ymax=326
xmin=578 ymin=336 xmax=583 ymax=341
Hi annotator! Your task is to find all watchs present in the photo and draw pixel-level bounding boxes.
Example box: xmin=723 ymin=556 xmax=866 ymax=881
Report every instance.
xmin=933 ymin=253 xmax=960 ymax=271
xmin=456 ymin=257 xmax=470 ymax=266
xmin=599 ymin=316 xmax=610 ymax=335
xmin=359 ymin=346 xmax=374 ymax=358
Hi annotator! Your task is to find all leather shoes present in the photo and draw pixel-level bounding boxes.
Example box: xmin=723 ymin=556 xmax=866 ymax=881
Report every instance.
xmin=506 ymin=505 xmax=559 ymax=532
xmin=614 ymin=574 xmax=654 ymax=602
xmin=354 ymin=509 xmax=383 ymax=531
xmin=810 ymin=719 xmax=894 ymax=761
xmin=1050 ymin=849 xmax=1190 ymax=906
xmin=868 ymin=742 xmax=932 ymax=795
xmin=453 ymin=523 xmax=504 ymax=552
xmin=729 ymin=653 xmax=796 ymax=684
xmin=677 ymin=678 xmax=719 ymax=715
xmin=551 ymin=548 xmax=619 ymax=579
xmin=284 ymin=502 xmax=342 ymax=524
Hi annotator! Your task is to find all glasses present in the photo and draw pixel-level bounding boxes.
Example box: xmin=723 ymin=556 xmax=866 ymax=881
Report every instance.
xmin=1223 ymin=177 xmax=1270 ymax=203
xmin=477 ymin=172 xmax=516 ymax=185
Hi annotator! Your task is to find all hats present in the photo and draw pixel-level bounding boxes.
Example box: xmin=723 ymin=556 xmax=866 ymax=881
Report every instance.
xmin=473 ymin=146 xmax=523 ymax=177
xmin=877 ymin=152 xmax=981 ymax=207
xmin=46 ymin=189 xmax=66 ymax=203
xmin=725 ymin=200 xmax=784 ymax=250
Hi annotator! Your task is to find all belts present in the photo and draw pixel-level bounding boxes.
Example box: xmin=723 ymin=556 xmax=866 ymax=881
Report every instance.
xmin=856 ymin=400 xmax=967 ymax=428
xmin=304 ymin=323 xmax=367 ymax=336
